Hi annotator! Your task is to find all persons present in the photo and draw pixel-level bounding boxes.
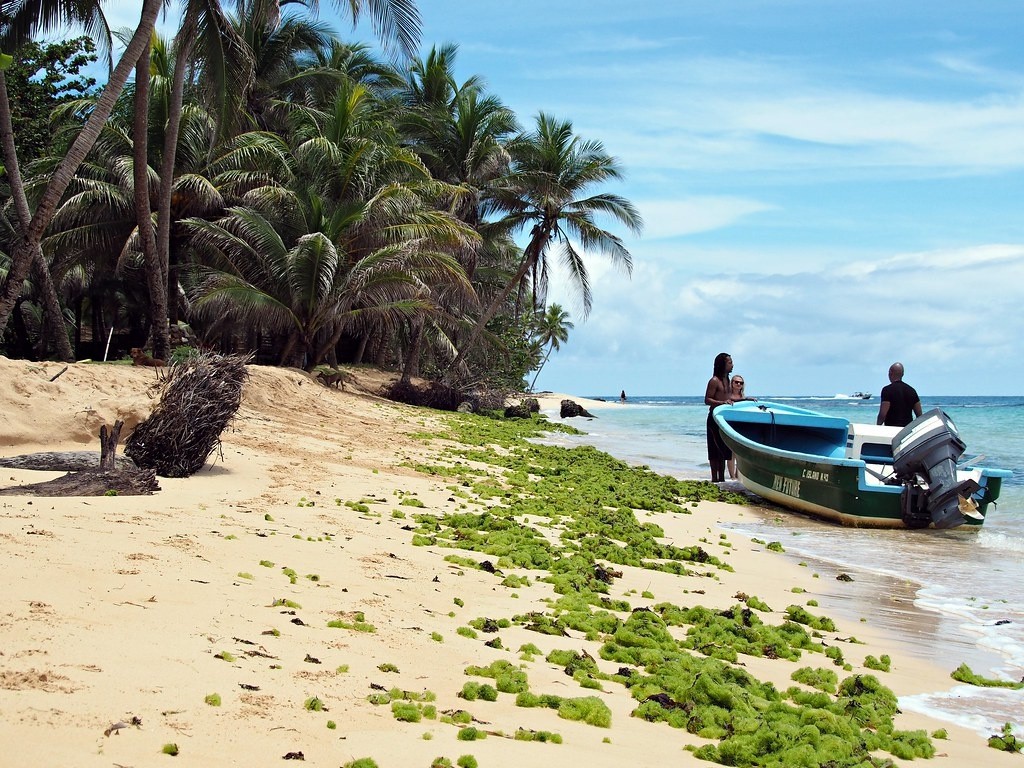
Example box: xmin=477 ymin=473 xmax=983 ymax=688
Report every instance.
xmin=705 ymin=353 xmax=757 ymax=482
xmin=621 ymin=390 xmax=625 ymax=405
xmin=727 ymin=375 xmax=745 ymax=478
xmin=876 ymin=362 xmax=922 ymax=427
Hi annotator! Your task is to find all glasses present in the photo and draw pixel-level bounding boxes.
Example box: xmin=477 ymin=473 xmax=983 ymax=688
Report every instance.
xmin=735 ymin=381 xmax=744 ymax=385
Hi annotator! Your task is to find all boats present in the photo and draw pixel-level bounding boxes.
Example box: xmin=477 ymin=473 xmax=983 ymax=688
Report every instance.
xmin=850 ymin=391 xmax=873 ymax=399
xmin=713 ymin=399 xmax=1015 ymax=533
